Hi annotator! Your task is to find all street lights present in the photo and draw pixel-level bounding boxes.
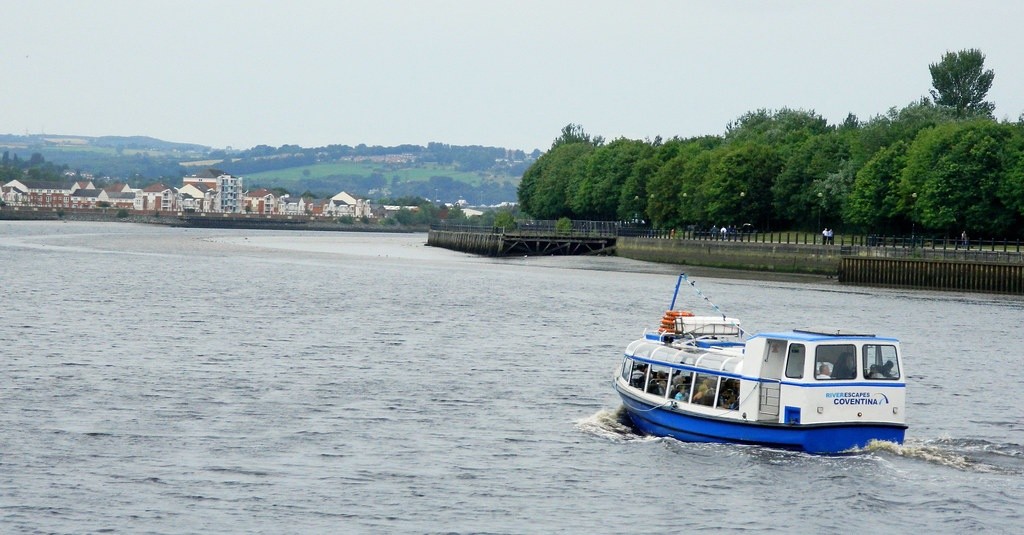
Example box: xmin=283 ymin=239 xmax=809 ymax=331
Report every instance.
xmin=739 ymin=192 xmax=745 ymax=221
xmin=912 ymin=192 xmax=917 ymax=221
xmin=635 ymin=196 xmax=639 ymax=205
xmin=817 ymin=193 xmax=822 ymax=235
xmin=683 ymin=193 xmax=687 ymax=205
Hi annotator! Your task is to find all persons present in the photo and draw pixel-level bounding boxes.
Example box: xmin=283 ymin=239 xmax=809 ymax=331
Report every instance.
xmin=829 ymin=352 xmax=855 ymax=379
xmin=961 ymin=230 xmax=967 ymax=247
xmin=827 ymin=229 xmax=834 ymax=244
xmin=628 ymin=362 xmax=742 ymax=412
xmin=822 ymin=228 xmax=828 ymax=245
xmin=710 ymin=224 xmax=734 ymax=240
xmin=864 ymin=360 xmax=893 ymax=379
xmin=815 ymin=364 xmax=831 ymax=381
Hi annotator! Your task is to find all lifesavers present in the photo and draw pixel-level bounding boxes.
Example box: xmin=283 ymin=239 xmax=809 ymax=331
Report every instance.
xmin=658 ymin=311 xmax=694 ymax=337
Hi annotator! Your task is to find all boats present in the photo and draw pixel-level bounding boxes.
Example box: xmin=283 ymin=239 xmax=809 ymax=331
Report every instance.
xmin=613 ymin=273 xmax=909 ymax=457
